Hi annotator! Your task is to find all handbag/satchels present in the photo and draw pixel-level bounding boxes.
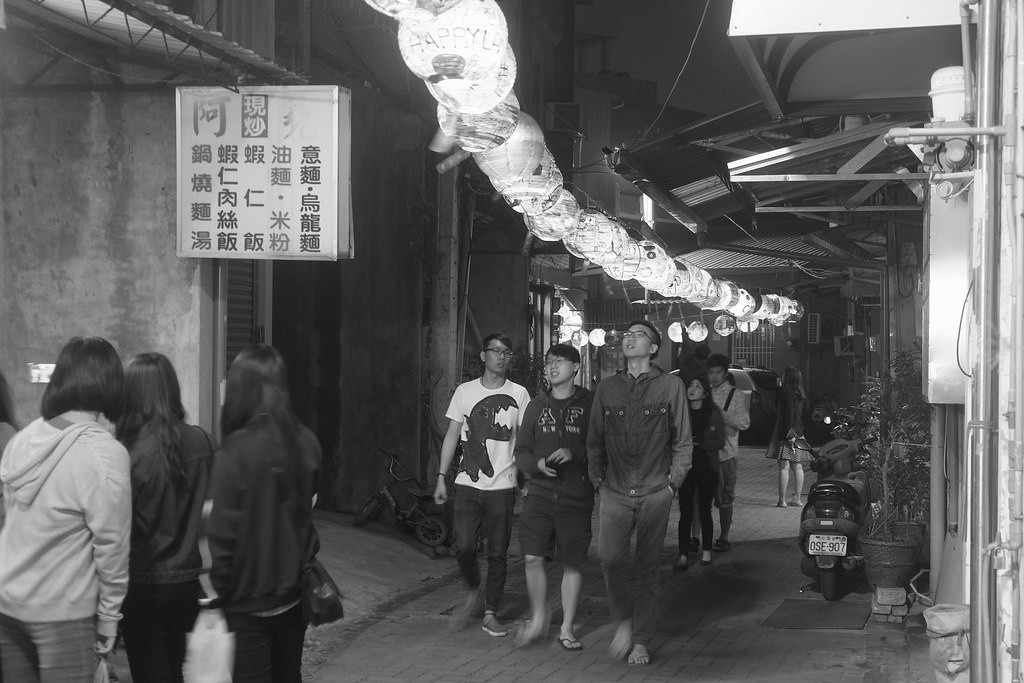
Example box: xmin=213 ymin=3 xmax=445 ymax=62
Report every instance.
xmin=298 ymin=556 xmax=346 ymax=630
xmin=182 ymin=605 xmax=237 ymax=683
xmin=679 ymin=468 xmax=697 ymax=493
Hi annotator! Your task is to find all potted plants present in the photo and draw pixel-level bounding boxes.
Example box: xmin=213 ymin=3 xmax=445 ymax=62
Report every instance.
xmin=833 ymin=350 xmax=929 ymax=592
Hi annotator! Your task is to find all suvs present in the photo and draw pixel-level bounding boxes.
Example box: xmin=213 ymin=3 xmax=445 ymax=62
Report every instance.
xmin=668 ymin=364 xmax=793 ymax=439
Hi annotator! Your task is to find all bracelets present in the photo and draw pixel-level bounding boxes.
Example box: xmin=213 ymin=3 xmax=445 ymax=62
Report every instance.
xmin=437 ymin=473 xmax=447 ymax=480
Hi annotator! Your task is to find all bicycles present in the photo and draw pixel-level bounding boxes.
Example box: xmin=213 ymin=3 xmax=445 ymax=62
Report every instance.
xmin=354 ymin=447 xmax=448 ymax=546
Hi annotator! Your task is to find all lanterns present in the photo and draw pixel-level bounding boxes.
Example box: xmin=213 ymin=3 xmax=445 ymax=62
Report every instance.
xmin=366 ymin=0 xmax=804 ymax=347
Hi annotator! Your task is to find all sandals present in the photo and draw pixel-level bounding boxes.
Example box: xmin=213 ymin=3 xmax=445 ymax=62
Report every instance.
xmin=689 ymin=537 xmax=700 ymax=552
xmin=713 ymin=539 xmax=732 ymax=552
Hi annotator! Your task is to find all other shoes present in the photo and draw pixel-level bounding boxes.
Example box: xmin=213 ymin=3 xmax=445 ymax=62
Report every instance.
xmin=787 ymin=497 xmax=803 ymax=506
xmin=677 ymin=560 xmax=689 ymax=572
xmin=777 ymin=502 xmax=787 ymax=508
xmin=700 ymin=552 xmax=712 ymax=566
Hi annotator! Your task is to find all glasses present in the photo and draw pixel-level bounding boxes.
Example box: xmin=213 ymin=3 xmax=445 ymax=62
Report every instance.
xmin=485 ymin=346 xmax=513 ymax=358
xmin=623 ymin=330 xmax=655 ymax=342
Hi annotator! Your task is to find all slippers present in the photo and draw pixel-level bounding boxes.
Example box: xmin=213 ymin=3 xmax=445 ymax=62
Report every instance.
xmin=558 ymin=638 xmax=582 ymax=650
xmin=481 ymin=617 xmax=507 ymax=636
xmin=628 ymin=650 xmax=651 ymax=666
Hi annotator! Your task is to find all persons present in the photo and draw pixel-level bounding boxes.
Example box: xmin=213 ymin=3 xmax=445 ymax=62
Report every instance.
xmin=114 ymin=353 xmax=219 ymax=683
xmin=512 ymin=343 xmax=596 ymax=652
xmin=207 ymin=343 xmax=323 ymax=683
xmin=765 ymin=366 xmax=815 ymax=508
xmin=673 ymin=353 xmax=751 ymax=571
xmin=434 ymin=333 xmax=531 ymax=637
xmin=0 ymin=335 xmax=132 ymax=683
xmin=586 ymin=321 xmax=692 ymax=667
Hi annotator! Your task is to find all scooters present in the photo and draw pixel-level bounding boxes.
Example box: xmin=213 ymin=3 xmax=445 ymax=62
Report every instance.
xmin=809 ymin=392 xmax=834 ymax=431
xmin=794 ymin=417 xmax=878 ymax=602
